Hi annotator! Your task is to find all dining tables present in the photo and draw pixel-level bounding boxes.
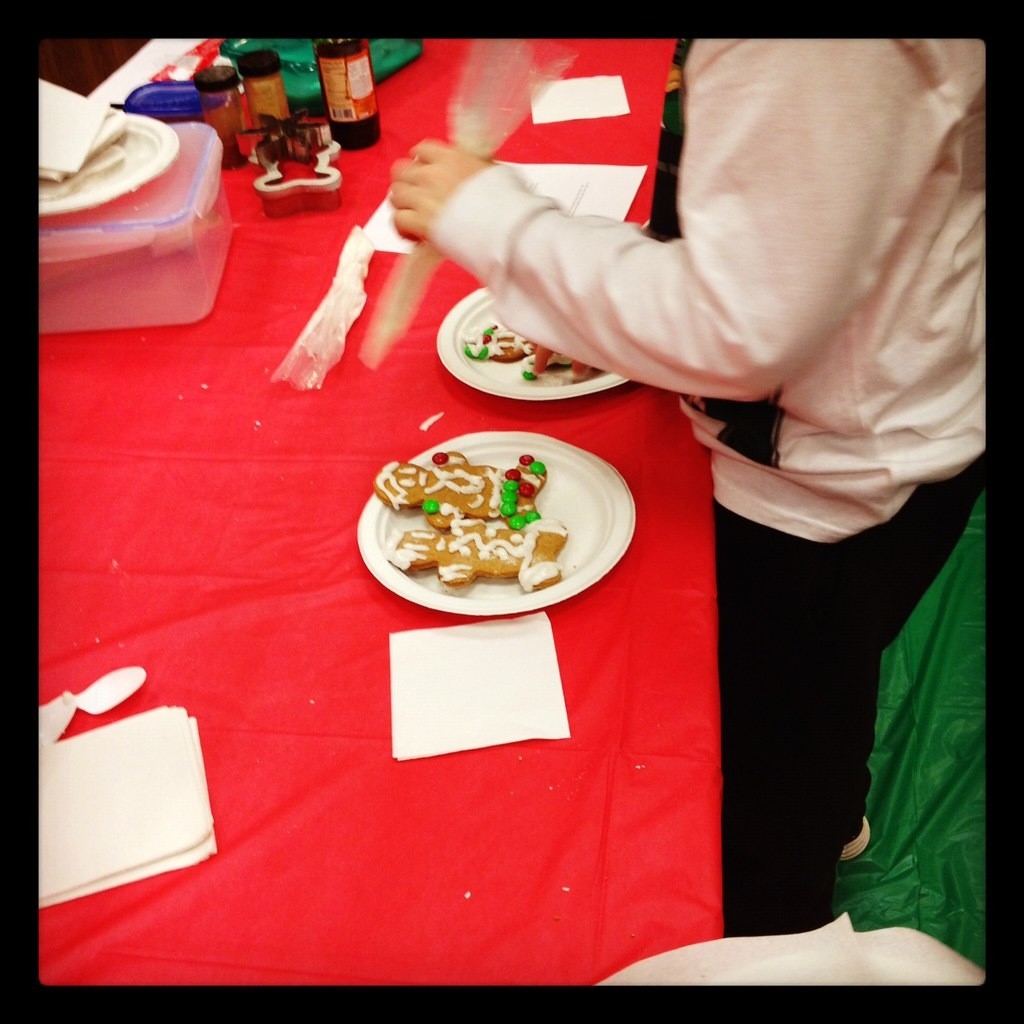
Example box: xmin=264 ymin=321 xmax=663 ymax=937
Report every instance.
xmin=37 ymin=31 xmax=720 ymax=980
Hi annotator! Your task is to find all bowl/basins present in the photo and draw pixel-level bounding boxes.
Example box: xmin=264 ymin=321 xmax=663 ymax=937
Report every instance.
xmin=39 ymin=121 xmax=230 ymax=336
xmin=220 ymin=40 xmax=423 ymax=116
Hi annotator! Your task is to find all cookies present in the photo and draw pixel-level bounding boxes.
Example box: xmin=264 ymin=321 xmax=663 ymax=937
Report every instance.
xmin=374 ymin=451 xmax=568 ymax=591
xmin=465 ymin=322 xmax=576 ymax=380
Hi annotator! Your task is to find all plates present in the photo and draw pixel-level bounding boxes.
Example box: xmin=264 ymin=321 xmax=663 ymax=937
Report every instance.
xmin=357 ymin=432 xmax=636 ymax=616
xmin=436 ymin=287 xmax=630 ymax=401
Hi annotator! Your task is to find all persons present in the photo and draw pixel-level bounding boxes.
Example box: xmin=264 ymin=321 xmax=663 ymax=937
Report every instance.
xmin=391 ymin=38 xmax=986 ymax=938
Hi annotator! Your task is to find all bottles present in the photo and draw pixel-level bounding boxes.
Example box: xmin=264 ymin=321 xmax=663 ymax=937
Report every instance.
xmin=235 ymin=49 xmax=292 ymax=129
xmin=193 ymin=65 xmax=251 ymax=171
xmin=311 ymin=39 xmax=382 ymax=152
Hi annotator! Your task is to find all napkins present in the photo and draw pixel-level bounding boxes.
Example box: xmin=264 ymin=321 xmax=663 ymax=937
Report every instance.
xmin=388 ymin=612 xmax=572 ymax=761
xmin=39 ymin=704 xmax=220 ymax=908
xmin=529 ymin=75 xmax=630 ymax=126
xmin=38 ymin=78 xmax=113 ymax=184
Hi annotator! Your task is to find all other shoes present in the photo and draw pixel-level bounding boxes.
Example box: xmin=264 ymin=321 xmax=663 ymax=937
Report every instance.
xmin=839 ymin=812 xmax=871 ymax=861
